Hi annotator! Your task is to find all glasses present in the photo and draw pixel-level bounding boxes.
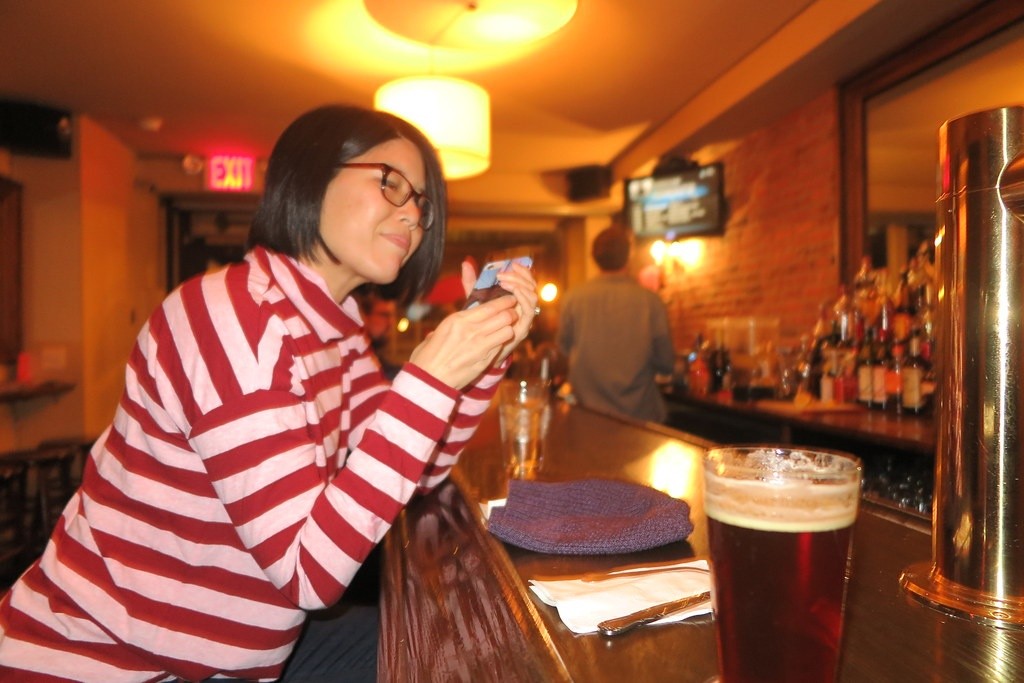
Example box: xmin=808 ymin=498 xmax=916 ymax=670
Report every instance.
xmin=340 ymin=163 xmax=434 ymax=231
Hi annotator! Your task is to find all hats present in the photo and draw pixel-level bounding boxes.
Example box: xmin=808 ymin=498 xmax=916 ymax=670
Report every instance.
xmin=486 ymin=477 xmax=693 ymax=554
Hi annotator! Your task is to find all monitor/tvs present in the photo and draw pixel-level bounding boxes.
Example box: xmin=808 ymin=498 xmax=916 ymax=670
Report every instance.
xmin=622 ymin=161 xmax=724 ymax=242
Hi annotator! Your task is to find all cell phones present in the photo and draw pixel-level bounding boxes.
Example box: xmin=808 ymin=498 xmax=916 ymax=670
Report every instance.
xmin=467 ymin=258 xmax=533 ymax=311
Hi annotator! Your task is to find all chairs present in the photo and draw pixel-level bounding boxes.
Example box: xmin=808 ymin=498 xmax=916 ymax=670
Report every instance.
xmin=0 ymin=435 xmax=96 ymax=591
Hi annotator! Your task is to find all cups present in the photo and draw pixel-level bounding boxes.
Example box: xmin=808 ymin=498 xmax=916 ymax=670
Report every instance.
xmin=498 ymin=379 xmax=554 ymax=480
xmin=702 ymin=447 xmax=862 ymax=683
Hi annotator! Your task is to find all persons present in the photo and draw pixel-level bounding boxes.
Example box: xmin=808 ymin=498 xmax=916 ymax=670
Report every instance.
xmin=1 ymin=103 xmax=541 ymax=682
xmin=556 ymin=226 xmax=674 ymax=423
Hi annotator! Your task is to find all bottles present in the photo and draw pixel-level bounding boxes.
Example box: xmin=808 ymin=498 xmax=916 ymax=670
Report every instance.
xmin=776 ymin=258 xmax=938 ymax=420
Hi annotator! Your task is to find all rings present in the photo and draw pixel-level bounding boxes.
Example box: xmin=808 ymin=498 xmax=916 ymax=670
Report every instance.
xmin=534 ymin=307 xmax=541 ymax=317
